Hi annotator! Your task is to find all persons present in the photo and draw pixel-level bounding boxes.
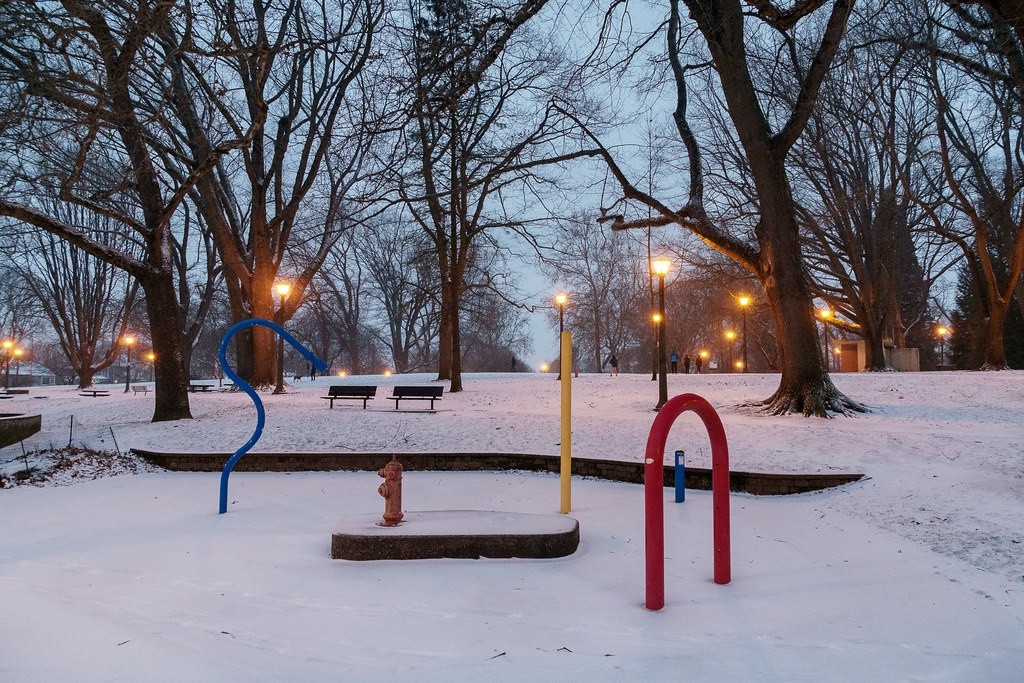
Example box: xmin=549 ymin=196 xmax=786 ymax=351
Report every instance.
xmin=510 ymin=357 xmax=515 ymax=372
xmin=610 ymin=355 xmax=618 ymax=377
xmin=696 ymin=355 xmax=702 ymax=374
xmin=685 ymin=355 xmax=690 ymax=374
xmin=311 ymin=365 xmax=316 ymax=381
xmin=670 ymin=351 xmax=678 ymax=373
xmin=306 ymin=363 xmax=310 ymax=377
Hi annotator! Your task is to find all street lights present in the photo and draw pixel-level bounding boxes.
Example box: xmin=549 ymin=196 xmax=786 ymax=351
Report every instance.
xmin=123 ymin=337 xmax=134 ymax=393
xmin=149 ymin=354 xmax=154 ymax=382
xmin=739 ymin=297 xmax=749 ymax=373
xmin=822 ymin=310 xmax=829 ymax=373
xmin=938 ymin=329 xmax=946 ymax=364
xmin=14 ymin=349 xmax=21 ymax=387
xmin=726 ymin=331 xmax=733 ymax=373
xmin=271 ymin=283 xmax=290 ymax=395
xmin=555 ymin=295 xmax=567 ymax=380
xmin=653 ymin=261 xmax=673 ymax=413
xmin=651 ymin=314 xmax=661 ymax=381
xmin=3 ymin=342 xmax=12 ymax=391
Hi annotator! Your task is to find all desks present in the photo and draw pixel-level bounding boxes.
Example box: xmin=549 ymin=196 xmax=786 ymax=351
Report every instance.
xmin=189 ymin=385 xmax=214 ymax=393
xmin=82 ymin=390 xmax=109 ymax=398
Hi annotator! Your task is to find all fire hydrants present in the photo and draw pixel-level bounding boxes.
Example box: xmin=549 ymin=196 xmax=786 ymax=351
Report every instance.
xmin=377 ymin=454 xmax=407 ymax=527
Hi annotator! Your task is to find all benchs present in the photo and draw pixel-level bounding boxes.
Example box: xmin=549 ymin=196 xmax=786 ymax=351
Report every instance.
xmin=386 ymin=385 xmax=445 ymax=410
xmin=131 ymin=385 xmax=152 ymax=396
xmin=321 ymin=386 xmax=377 ymax=410
xmin=6 ymin=390 xmax=30 ymax=395
xmin=187 ymin=390 xmax=219 ymax=393
xmin=78 ymin=394 xmax=111 ymax=396
